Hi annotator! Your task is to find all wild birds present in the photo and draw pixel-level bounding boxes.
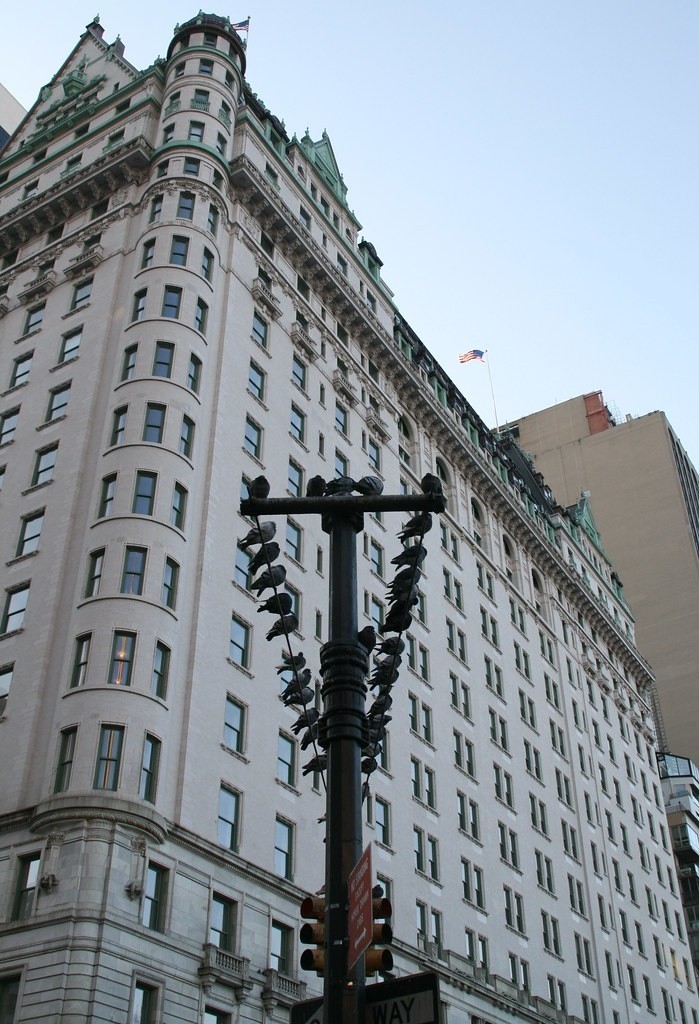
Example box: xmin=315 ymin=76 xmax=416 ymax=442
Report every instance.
xmin=370 ymin=884 xmax=384 ymax=899
xmin=280 ymin=668 xmax=327 ymax=777
xmin=248 ymin=474 xmax=271 ymax=499
xmin=359 ymin=625 xmax=377 ymax=656
xmin=248 ymin=564 xmax=288 ymax=597
xmin=305 ymin=475 xmax=384 ymax=497
xmin=379 ymin=567 xmax=422 ymax=636
xmin=316 ymin=884 xmax=325 ymax=896
xmin=265 ymin=610 xmax=299 ymax=642
xmin=275 ymin=652 xmax=306 ymax=675
xmin=361 ymin=636 xmax=406 ymax=808
xmin=396 ymin=513 xmax=433 ymax=544
xmin=317 ymin=812 xmax=326 ymax=824
xmin=238 ymin=521 xmax=277 ymax=551
xmin=390 ymin=545 xmax=428 ymax=571
xmin=246 ymin=542 xmax=280 ymax=576
xmin=256 ymin=593 xmax=292 ymax=616
xmin=421 ymin=471 xmax=444 ymax=501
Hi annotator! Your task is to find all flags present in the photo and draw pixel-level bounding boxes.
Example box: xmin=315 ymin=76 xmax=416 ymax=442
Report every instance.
xmin=460 ymin=350 xmax=484 ymax=363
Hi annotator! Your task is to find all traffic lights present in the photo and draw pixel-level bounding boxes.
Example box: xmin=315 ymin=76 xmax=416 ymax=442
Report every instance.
xmin=365 ymin=896 xmax=394 ymax=976
xmin=299 ymin=896 xmax=326 ymax=978
